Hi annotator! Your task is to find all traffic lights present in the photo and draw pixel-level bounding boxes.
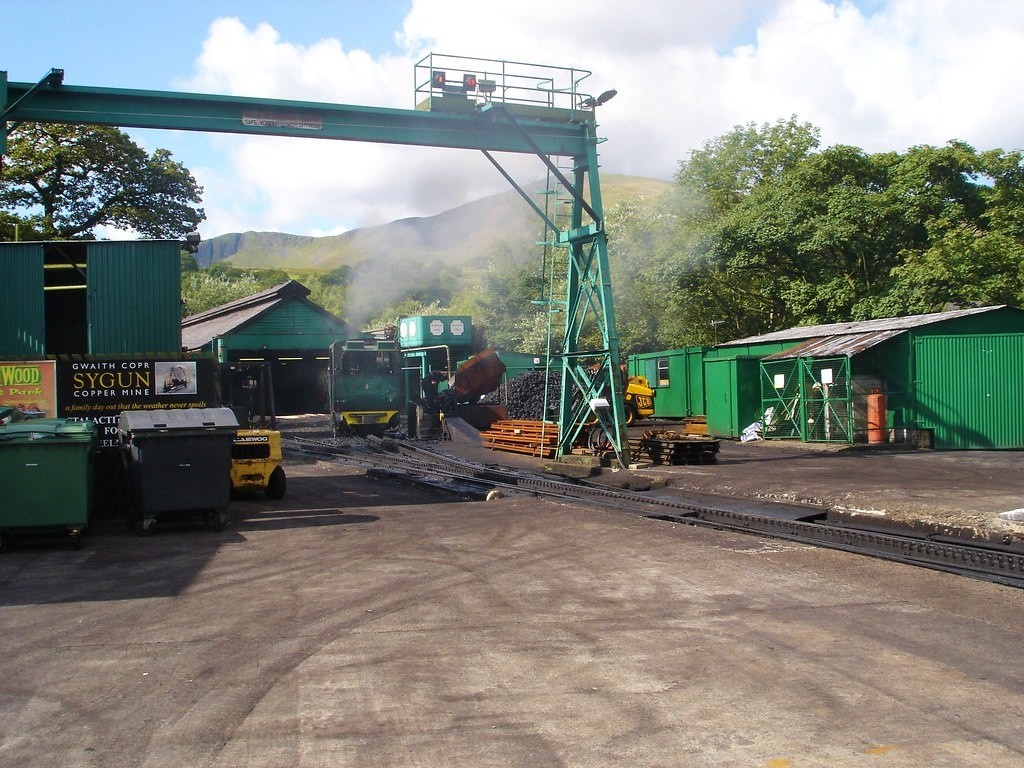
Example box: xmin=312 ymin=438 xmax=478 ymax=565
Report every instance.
xmin=433 ymin=70 xmax=445 ymax=87
xmin=464 ymin=73 xmax=478 ymax=92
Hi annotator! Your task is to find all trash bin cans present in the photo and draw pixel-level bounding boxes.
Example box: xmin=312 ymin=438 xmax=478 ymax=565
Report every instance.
xmin=118 ymin=408 xmax=240 ymax=532
xmin=0 ymin=406 xmax=96 ymax=550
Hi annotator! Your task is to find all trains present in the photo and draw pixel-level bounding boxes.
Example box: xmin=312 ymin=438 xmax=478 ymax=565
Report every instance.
xmin=329 ymin=337 xmax=402 ymax=439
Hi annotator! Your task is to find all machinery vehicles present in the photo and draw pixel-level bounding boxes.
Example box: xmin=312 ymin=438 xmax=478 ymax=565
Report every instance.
xmin=569 ymin=360 xmax=658 ymax=426
xmin=218 ymin=360 xmax=289 ymax=503
